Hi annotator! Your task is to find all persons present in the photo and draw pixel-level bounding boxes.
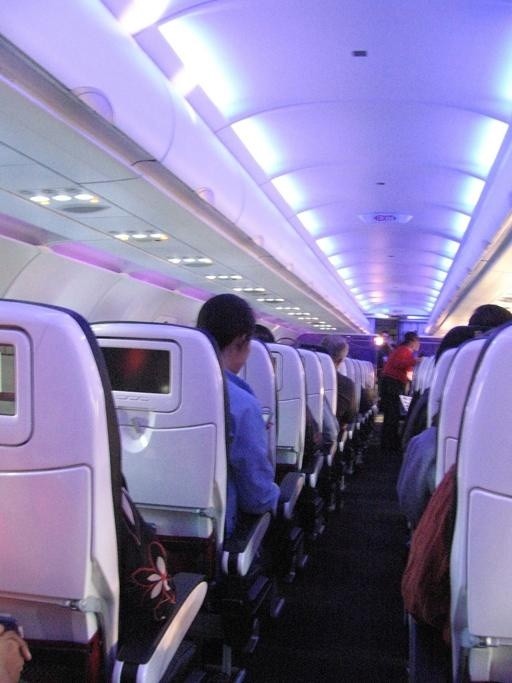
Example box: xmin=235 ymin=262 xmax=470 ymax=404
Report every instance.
xmin=402 ymin=326 xmax=474 ymax=448
xmin=319 ymin=334 xmax=357 ymax=428
xmin=118 ymin=477 xmax=177 ymax=639
xmin=467 ymin=303 xmax=512 ymax=338
xmin=377 ymin=331 xmax=420 ymax=463
xmin=194 ymin=292 xmax=294 ymax=575
xmin=0 ymin=613 xmax=32 ymax=682
xmin=252 ymin=324 xmax=324 ymax=456
xmin=399 ymin=461 xmax=454 ymax=682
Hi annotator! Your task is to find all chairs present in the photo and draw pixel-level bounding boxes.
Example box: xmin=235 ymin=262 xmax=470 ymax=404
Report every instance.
xmin=401 ymin=325 xmax=511 ymax=683
xmin=80 ymin=315 xmax=275 ymax=680
xmin=2 ymin=299 xmax=247 ymax=682
xmin=217 ymin=329 xmax=378 ymax=553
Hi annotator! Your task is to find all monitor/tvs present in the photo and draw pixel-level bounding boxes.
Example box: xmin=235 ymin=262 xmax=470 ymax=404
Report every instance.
xmin=95 ymin=338 xmax=181 ymax=413
xmin=270 ymin=352 xmax=282 ymax=391
xmin=0 ymin=328 xmax=32 ymax=446
